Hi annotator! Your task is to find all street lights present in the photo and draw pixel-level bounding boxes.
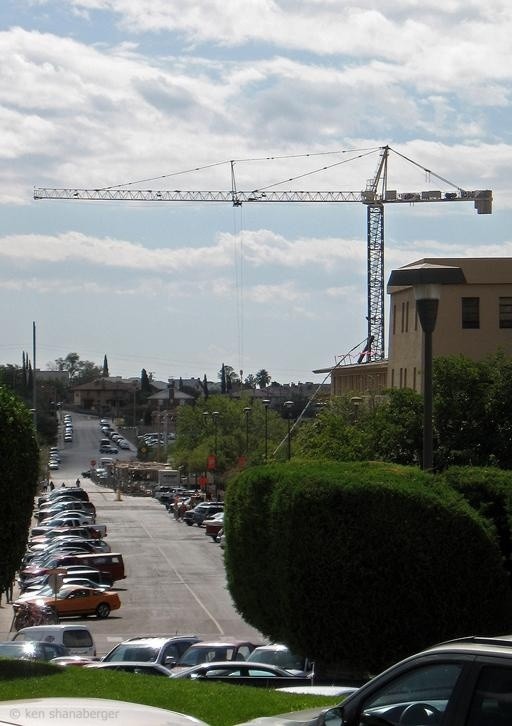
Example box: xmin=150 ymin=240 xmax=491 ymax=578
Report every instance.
xmin=261 ymin=398 xmax=272 ymax=463
xmin=202 ymin=411 xmax=222 ymax=484
xmin=283 ymin=400 xmax=295 ymax=462
xmin=387 ymin=261 xmax=470 ymax=472
xmin=242 ymin=406 xmax=253 ymax=466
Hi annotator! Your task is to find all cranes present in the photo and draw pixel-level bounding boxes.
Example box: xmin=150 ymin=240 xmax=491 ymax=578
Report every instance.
xmin=17 ymin=145 xmax=494 ymax=362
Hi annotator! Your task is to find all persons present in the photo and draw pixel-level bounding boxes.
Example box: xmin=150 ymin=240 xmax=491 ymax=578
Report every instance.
xmin=50 ymin=481 xmax=54 ymax=490
xmin=76 ymin=479 xmax=80 ymax=487
xmin=62 ymin=483 xmax=65 ymax=487
xmin=43 ymin=478 xmax=48 ymax=493
xmin=181 ymin=502 xmax=187 ymax=519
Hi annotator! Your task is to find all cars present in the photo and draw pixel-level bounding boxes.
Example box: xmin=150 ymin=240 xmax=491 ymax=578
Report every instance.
xmin=46 ymin=630 xmax=309 ymax=676
xmin=63 ymin=413 xmax=74 ymax=443
xmin=80 ymin=456 xmax=115 ymax=488
xmin=135 ymin=431 xmax=176 ymax=450
xmin=98 ymin=418 xmax=130 ymax=454
xmin=154 ymin=483 xmax=226 ymax=549
xmin=229 ymin=628 xmax=511 ymax=726
xmin=47 ymin=446 xmax=61 ymax=471
xmin=12 ymin=485 xmax=127 ymax=619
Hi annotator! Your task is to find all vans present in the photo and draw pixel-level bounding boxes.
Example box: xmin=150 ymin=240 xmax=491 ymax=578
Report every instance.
xmin=0 ymin=624 xmax=97 ymax=664
